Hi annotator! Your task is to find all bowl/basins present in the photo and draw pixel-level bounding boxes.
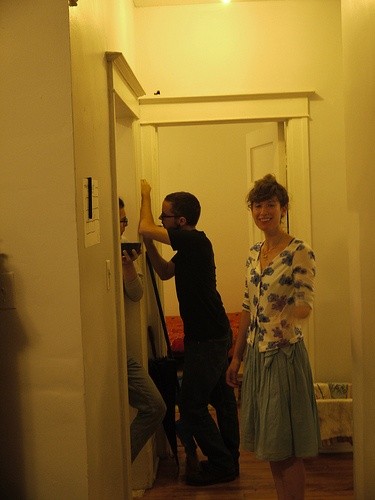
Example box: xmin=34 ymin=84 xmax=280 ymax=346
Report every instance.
xmin=121 ymin=243 xmax=141 ymax=257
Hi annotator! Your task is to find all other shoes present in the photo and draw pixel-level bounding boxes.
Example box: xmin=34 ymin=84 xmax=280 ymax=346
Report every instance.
xmin=200 ymin=459 xmax=239 ymax=477
xmin=185 ymin=466 xmax=235 ymax=485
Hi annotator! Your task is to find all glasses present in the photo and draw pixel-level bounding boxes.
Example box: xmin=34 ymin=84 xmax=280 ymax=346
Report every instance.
xmin=160 ymin=212 xmax=175 ymax=219
xmin=120 ymin=219 xmax=127 ymax=226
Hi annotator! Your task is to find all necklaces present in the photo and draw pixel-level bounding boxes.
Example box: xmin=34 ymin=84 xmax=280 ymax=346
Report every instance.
xmin=263 ymin=230 xmax=285 ymax=260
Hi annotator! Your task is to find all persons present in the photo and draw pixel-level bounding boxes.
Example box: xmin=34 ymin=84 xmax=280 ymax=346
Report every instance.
xmin=225 ymin=175 xmax=322 ymax=500
xmin=119 ymin=198 xmax=167 ymax=465
xmin=138 ymin=177 xmax=241 ymax=485
